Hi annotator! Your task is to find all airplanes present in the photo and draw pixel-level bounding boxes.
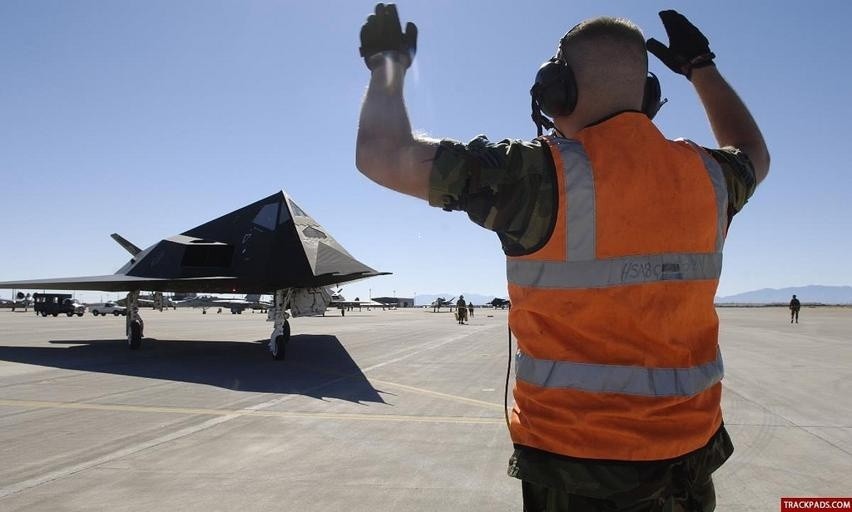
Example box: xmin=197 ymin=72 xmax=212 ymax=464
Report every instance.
xmin=0 ymin=292 xmax=32 ymax=312
xmin=0 ymin=190 xmax=393 ymax=361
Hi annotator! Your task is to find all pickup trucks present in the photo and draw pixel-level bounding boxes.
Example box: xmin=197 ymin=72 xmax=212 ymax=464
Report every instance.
xmin=89 ymin=301 xmax=127 ymax=315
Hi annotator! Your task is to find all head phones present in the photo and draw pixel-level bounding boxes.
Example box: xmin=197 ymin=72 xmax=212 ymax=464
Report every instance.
xmin=530 ymin=23 xmax=668 ymax=120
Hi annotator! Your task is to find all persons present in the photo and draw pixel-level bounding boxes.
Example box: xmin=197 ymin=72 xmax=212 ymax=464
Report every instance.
xmin=456 ymin=295 xmax=466 ymax=324
xmin=467 ymin=302 xmax=474 ymax=318
xmin=788 ymin=295 xmax=800 ymax=324
xmin=353 ymin=2 xmax=771 ymax=511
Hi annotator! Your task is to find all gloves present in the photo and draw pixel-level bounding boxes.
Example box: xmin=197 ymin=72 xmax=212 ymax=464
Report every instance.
xmin=645 ymin=8 xmax=718 ymax=82
xmin=357 ymin=2 xmax=419 ymax=71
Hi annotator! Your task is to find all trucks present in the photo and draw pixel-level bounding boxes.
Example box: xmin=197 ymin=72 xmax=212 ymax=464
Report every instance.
xmin=33 ymin=293 xmax=86 ymax=316
